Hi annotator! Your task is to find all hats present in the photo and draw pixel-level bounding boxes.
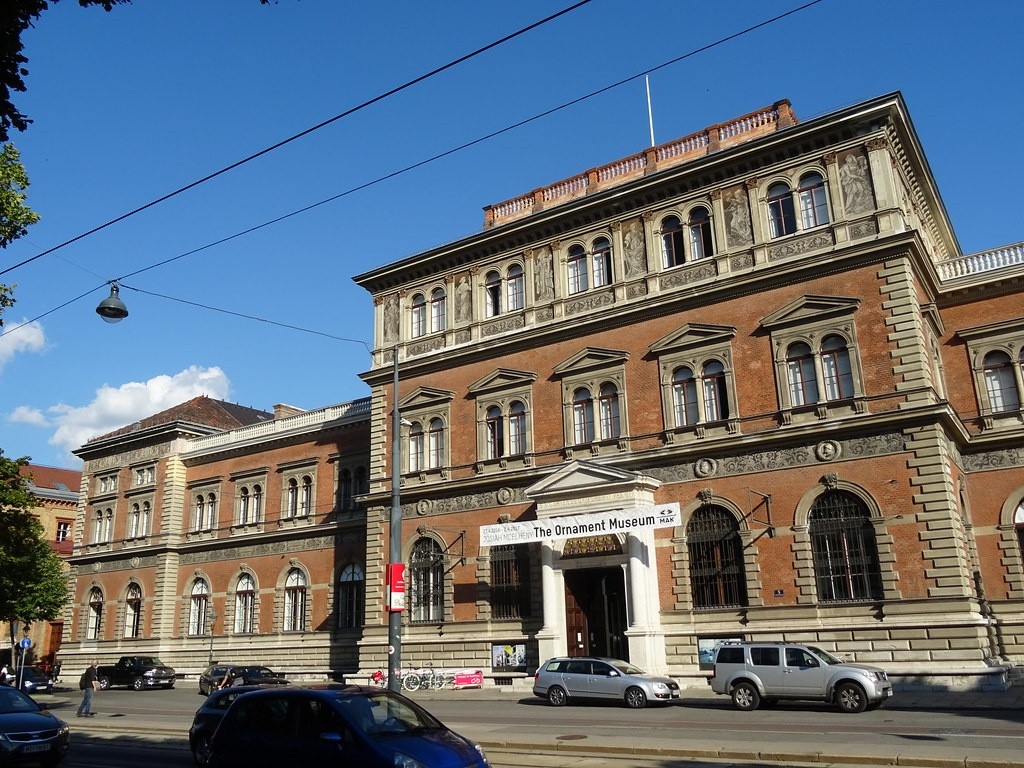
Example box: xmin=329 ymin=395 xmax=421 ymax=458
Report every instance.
xmin=226 ymin=665 xmax=232 ymax=669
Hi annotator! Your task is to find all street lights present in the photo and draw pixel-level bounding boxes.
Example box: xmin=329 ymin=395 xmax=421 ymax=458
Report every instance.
xmin=209 ymin=612 xmax=218 ymax=667
xmin=19 ymin=623 xmax=31 ymax=690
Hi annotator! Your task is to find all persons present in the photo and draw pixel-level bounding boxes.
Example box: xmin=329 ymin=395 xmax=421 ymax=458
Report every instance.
xmin=346 ymin=696 xmax=381 ymax=742
xmin=217 ymin=663 xmax=244 ymax=690
xmin=76 ymin=660 xmax=99 ymax=718
xmin=0 ymin=664 xmax=17 ymax=685
xmin=46 ymin=661 xmax=63 ymax=695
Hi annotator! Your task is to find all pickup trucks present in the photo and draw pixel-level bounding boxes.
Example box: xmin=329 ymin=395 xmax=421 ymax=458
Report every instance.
xmin=97 ymin=656 xmax=177 ymax=691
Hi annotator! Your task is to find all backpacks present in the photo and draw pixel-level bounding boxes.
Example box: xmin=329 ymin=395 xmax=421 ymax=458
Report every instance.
xmin=79 ymin=673 xmax=86 ymax=690
xmin=4 ymin=666 xmax=15 ymax=675
xmin=230 ymin=669 xmax=242 ymax=678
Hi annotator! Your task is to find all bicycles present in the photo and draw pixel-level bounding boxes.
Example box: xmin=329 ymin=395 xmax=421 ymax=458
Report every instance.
xmin=401 ymin=662 xmax=446 ymax=691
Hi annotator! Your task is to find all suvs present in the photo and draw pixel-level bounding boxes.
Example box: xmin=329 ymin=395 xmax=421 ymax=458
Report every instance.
xmin=706 ymin=641 xmax=893 ymax=713
xmin=532 ymin=655 xmax=681 ymax=709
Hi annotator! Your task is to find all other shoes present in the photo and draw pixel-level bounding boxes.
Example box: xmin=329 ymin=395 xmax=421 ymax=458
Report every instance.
xmin=77 ymin=712 xmax=84 ymax=716
xmin=85 ymin=714 xmax=94 ymax=717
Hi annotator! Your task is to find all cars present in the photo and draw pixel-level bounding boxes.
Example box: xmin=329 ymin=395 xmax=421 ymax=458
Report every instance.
xmin=204 ymin=685 xmax=492 ymax=768
xmin=229 ymin=666 xmax=291 ymax=686
xmin=189 ymin=685 xmax=285 ymax=768
xmin=15 ymin=666 xmax=53 ymax=694
xmin=0 ymin=683 xmax=70 ymax=768
xmin=199 ymin=664 xmax=240 ymax=696
